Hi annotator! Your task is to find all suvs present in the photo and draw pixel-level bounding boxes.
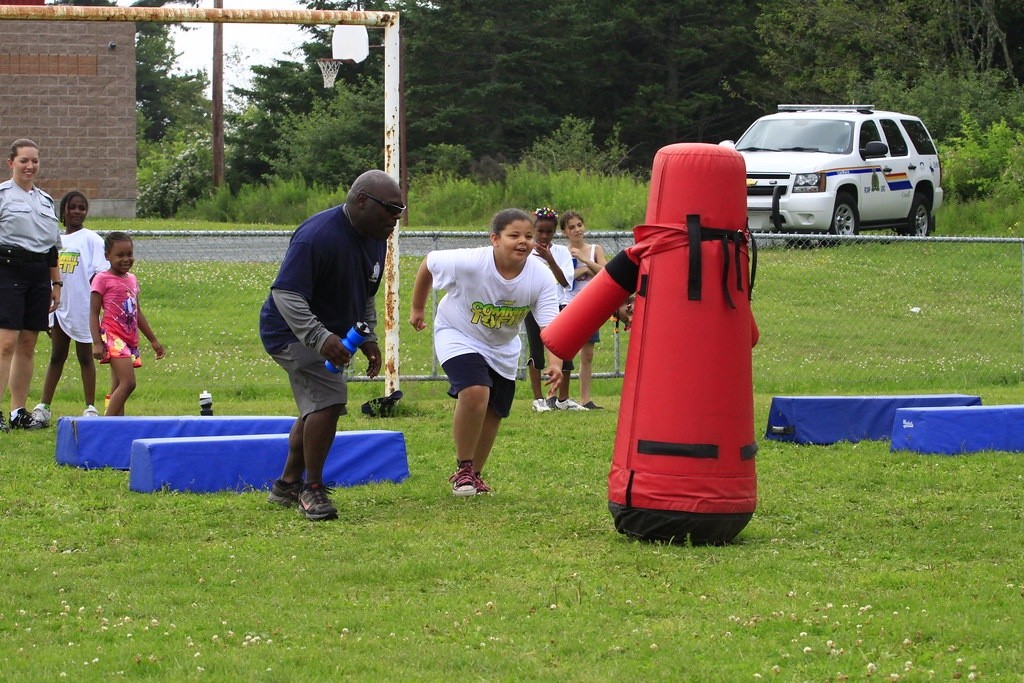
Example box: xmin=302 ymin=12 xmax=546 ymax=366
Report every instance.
xmin=730 ymin=103 xmax=945 ymax=248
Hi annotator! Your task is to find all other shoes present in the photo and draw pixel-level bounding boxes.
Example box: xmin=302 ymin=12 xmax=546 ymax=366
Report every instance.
xmin=583 ymin=400 xmax=605 ymax=409
xmin=546 ymin=395 xmax=558 ymax=409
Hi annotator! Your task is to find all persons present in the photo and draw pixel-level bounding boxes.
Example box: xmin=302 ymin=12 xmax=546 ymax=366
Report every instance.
xmin=546 ymin=211 xmax=606 ymax=409
xmin=259 ymin=170 xmax=404 ymax=521
xmin=611 ymin=297 xmax=635 ymax=333
xmin=0 ymin=139 xmax=63 ymax=431
xmin=32 ymin=192 xmax=111 ymax=422
xmin=524 ymin=208 xmax=589 ymax=412
xmin=89 ymin=232 xmax=165 ymax=416
xmin=410 ymin=208 xmax=563 ymax=496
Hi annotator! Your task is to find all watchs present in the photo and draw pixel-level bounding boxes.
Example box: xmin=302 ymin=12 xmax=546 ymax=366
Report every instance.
xmin=52 ymin=281 xmax=63 ymax=287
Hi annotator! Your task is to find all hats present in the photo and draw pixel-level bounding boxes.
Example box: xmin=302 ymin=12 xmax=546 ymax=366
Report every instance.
xmin=361 ymin=390 xmax=404 ymax=416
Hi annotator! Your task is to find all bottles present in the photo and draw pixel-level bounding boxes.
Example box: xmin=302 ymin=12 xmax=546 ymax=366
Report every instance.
xmin=569 ymin=256 xmax=577 ymax=292
xmin=199 ymin=391 xmax=213 ymax=416
xmin=324 ymin=321 xmax=370 ymax=374
xmin=104 ymin=395 xmax=110 ymax=415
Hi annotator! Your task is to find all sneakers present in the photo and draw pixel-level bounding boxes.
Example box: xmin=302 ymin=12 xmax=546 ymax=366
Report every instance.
xmin=532 ymin=397 xmax=550 ymax=411
xmin=31 ymin=404 xmax=50 ymax=421
xmin=8 ymin=407 xmax=49 ymax=430
xmin=449 ymin=462 xmax=476 ymax=495
xmin=269 ymin=478 xmax=303 ymax=506
xmin=555 ymin=399 xmax=589 ymax=411
xmin=473 ymin=472 xmax=491 ymax=494
xmin=298 ymin=480 xmax=336 ymax=518
xmin=83 ymin=404 xmax=98 ymax=416
xmin=0 ymin=411 xmax=8 ymax=432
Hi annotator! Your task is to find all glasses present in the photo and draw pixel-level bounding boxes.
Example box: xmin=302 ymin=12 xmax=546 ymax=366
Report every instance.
xmin=356 ymin=190 xmax=407 ymax=215
